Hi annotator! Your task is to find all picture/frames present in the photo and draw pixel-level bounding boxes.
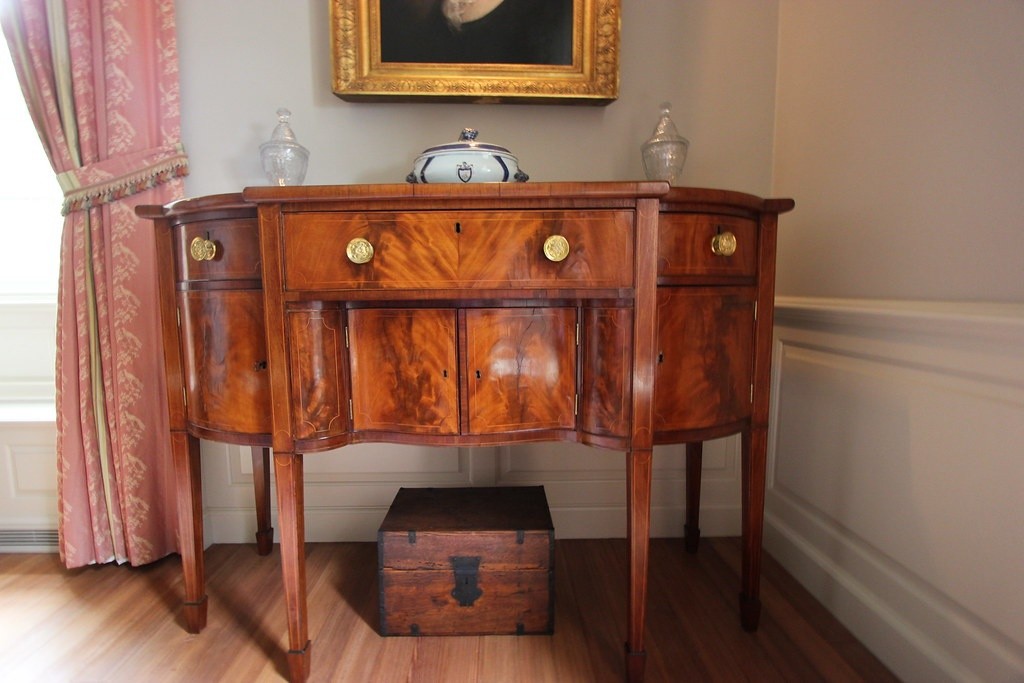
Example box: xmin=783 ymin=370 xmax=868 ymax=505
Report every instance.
xmin=328 ymin=0 xmax=621 ymax=107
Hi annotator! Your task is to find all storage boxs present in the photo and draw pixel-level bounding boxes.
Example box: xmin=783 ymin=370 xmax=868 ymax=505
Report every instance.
xmin=379 ymin=486 xmax=556 ymax=637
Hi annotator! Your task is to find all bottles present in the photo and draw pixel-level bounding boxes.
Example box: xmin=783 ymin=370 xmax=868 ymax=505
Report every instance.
xmin=259 ymin=104 xmax=311 ymax=186
xmin=638 ymin=100 xmax=695 ymax=186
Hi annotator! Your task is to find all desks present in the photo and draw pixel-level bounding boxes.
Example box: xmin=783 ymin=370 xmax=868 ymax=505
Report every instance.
xmin=133 ymin=180 xmax=794 ymax=683
